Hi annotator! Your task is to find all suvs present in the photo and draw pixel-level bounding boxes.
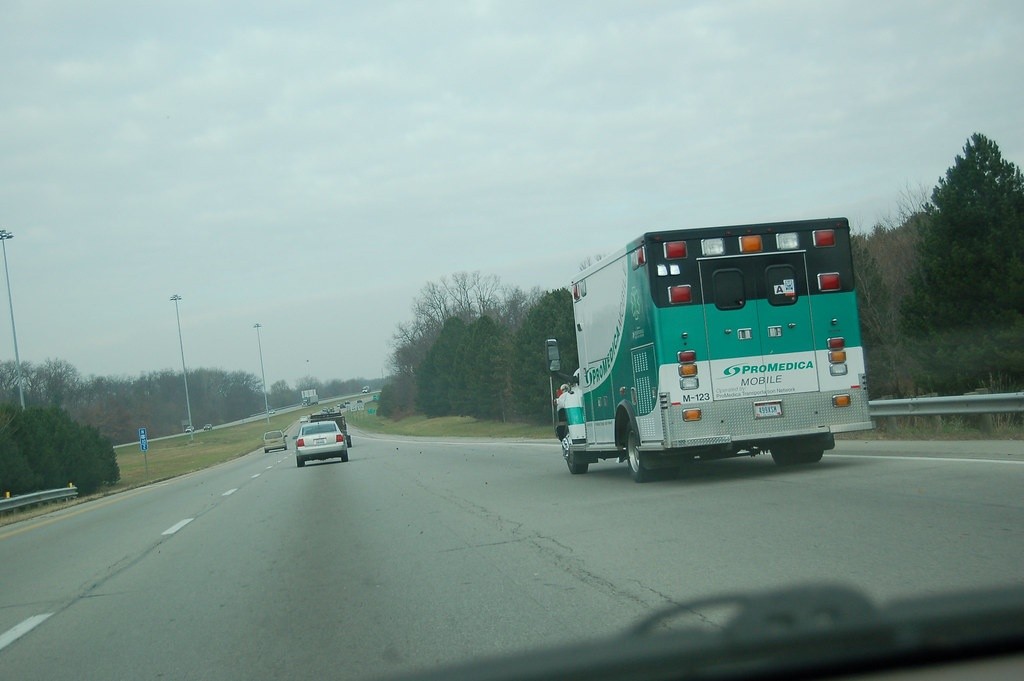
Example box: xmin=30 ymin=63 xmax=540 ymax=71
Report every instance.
xmin=262 ymin=430 xmax=288 ymax=453
xmin=309 ymin=412 xmax=352 ymax=448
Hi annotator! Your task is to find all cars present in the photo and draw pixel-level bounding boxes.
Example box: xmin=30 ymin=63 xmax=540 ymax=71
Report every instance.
xmin=292 ymin=421 xmax=350 ymax=467
xmin=203 ymin=423 xmax=212 ymax=431
xmin=322 ymin=400 xmax=363 ymax=413
xmin=269 ymin=409 xmax=276 ymax=414
xmin=185 ymin=425 xmax=196 ymax=433
xmin=299 ymin=415 xmax=309 ymax=424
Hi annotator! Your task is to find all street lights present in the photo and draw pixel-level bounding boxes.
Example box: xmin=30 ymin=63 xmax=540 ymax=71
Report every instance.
xmin=0 ymin=229 xmax=27 ymax=411
xmin=170 ymin=293 xmax=194 ymax=434
xmin=253 ymin=322 xmax=271 ymax=424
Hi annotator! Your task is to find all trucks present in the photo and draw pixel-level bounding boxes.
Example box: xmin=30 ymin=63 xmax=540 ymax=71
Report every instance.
xmin=544 ymin=218 xmax=878 ymax=483
xmin=301 ymin=394 xmax=319 ymax=407
xmin=362 ymin=386 xmax=370 ymax=394
